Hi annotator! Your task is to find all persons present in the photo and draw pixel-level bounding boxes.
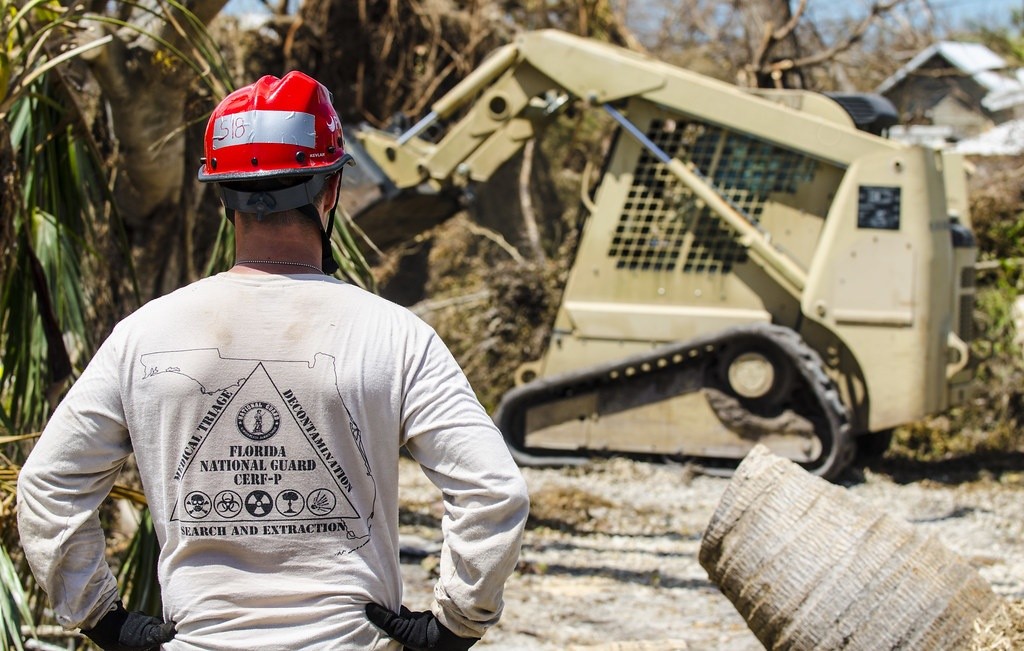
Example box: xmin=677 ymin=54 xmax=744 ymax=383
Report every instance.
xmin=17 ymin=71 xmax=531 ymax=651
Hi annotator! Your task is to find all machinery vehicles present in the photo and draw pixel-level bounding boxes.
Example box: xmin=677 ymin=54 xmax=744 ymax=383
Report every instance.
xmin=335 ymin=26 xmax=980 ymax=489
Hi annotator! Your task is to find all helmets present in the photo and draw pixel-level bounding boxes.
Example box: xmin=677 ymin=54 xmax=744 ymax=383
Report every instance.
xmin=197 ymin=71 xmax=356 ymax=183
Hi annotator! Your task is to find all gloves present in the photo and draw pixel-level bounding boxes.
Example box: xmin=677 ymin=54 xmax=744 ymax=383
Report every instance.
xmin=366 ymin=603 xmax=481 ymax=651
xmin=79 ymin=600 xmax=178 ymax=651
